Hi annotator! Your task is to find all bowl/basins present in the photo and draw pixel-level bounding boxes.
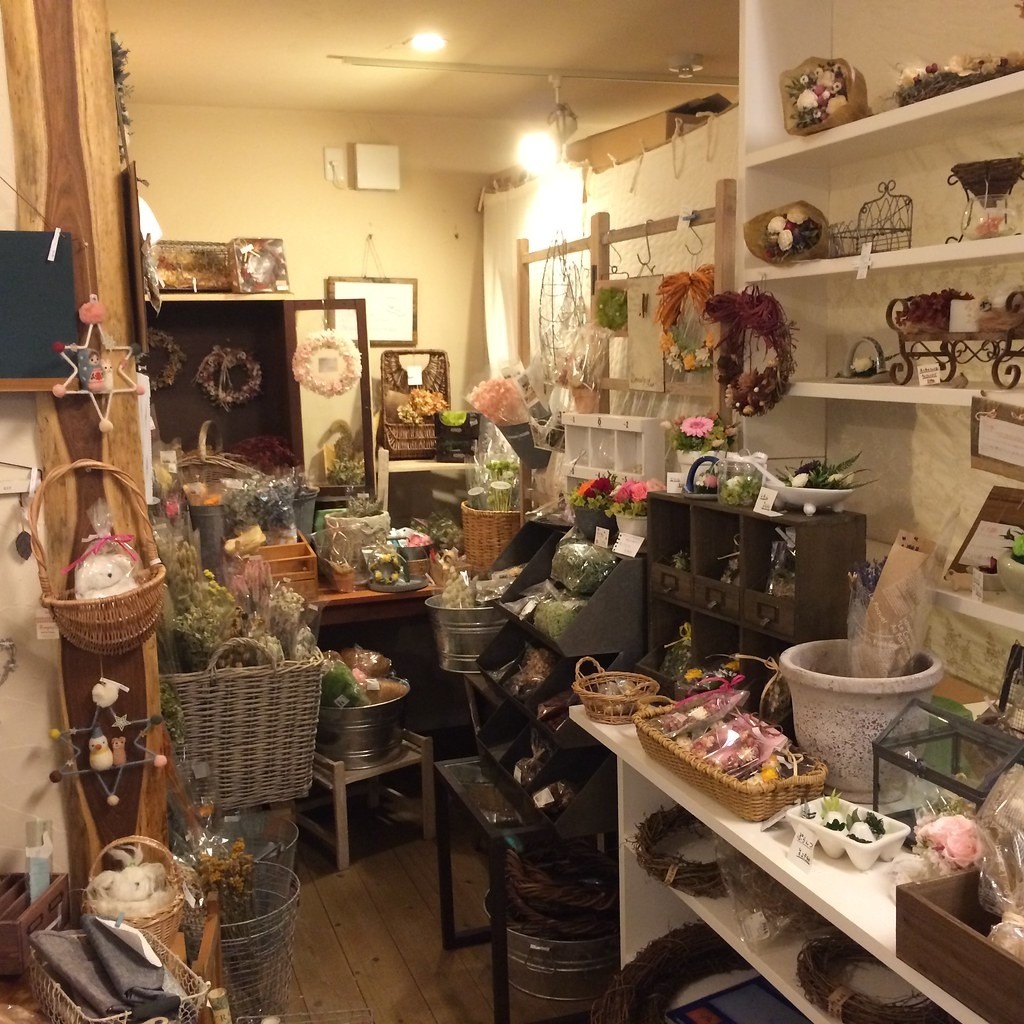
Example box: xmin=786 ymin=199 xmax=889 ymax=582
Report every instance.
xmin=427 ymin=594 xmax=514 ymax=673
xmin=314 ymin=677 xmax=409 ymax=771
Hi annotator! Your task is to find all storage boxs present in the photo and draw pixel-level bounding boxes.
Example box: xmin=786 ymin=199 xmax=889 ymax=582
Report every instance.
xmin=0 ymin=871 xmax=70 ymax=976
xmin=895 ymin=871 xmax=1024 ymax=1023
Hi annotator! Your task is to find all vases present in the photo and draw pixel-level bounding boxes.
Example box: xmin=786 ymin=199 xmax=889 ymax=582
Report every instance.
xmin=675 ymin=448 xmax=738 ymax=478
xmin=615 ymin=514 xmax=647 ymax=555
xmin=571 ymin=509 xmax=613 ymax=541
xmin=776 ymin=640 xmax=943 ymax=810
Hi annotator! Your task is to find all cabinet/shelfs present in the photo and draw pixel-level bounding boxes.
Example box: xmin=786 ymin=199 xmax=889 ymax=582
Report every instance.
xmin=569 ymin=703 xmax=990 ymax=1023
xmin=646 ymin=491 xmax=867 ymax=685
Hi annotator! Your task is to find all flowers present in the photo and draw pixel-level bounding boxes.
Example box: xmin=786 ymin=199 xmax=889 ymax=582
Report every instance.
xmin=895 ymin=289 xmax=974 ymax=329
xmin=659 ymin=268 xmax=713 ymax=371
xmin=370 ymin=552 xmax=401 ymax=583
xmin=914 ymin=808 xmax=979 ymax=870
xmin=849 ymin=537 xmax=922 ymax=602
xmin=293 ymin=330 xmax=362 ymax=396
xmin=399 ymin=388 xmax=447 ymax=424
xmin=764 ymin=207 xmax=815 ymax=252
xmin=195 ymin=346 xmax=263 ymax=405
xmin=617 ymin=480 xmax=667 ymax=513
xmin=657 ymin=408 xmax=740 ymax=459
xmin=709 ymin=291 xmax=794 ymax=419
xmin=573 ymin=477 xmax=615 ymax=507
xmin=134 ymin=328 xmax=185 ymax=391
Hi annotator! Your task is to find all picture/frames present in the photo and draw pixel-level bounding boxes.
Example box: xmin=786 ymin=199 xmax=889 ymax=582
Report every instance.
xmin=326 ymin=275 xmax=419 ymax=346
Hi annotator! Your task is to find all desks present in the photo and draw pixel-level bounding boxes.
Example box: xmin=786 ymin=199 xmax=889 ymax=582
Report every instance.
xmin=430 ymin=756 xmax=619 ymax=1024
xmin=296 ymin=728 xmax=435 ymax=872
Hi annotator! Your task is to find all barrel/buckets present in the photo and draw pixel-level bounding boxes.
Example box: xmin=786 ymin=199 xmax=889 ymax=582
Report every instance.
xmin=482 ymin=887 xmax=619 ymax=1024
xmin=200 ymin=812 xmax=298 ymax=892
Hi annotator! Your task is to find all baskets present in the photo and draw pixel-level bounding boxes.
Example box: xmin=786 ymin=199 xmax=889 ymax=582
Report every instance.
xmin=28 ymin=458 xmax=167 ymax=658
xmin=87 ymin=835 xmax=188 ymax=953
xmin=573 ymin=656 xmax=660 ymax=725
xmin=381 ymin=348 xmax=453 ymax=459
xmin=175 ymin=421 xmax=266 ymax=484
xmin=460 ymin=498 xmax=522 ymax=580
xmin=160 ymin=636 xmax=325 ymax=815
xmin=631 ymin=696 xmax=828 ymax=821
xmin=26 ymin=927 xmax=212 ymax=1024
